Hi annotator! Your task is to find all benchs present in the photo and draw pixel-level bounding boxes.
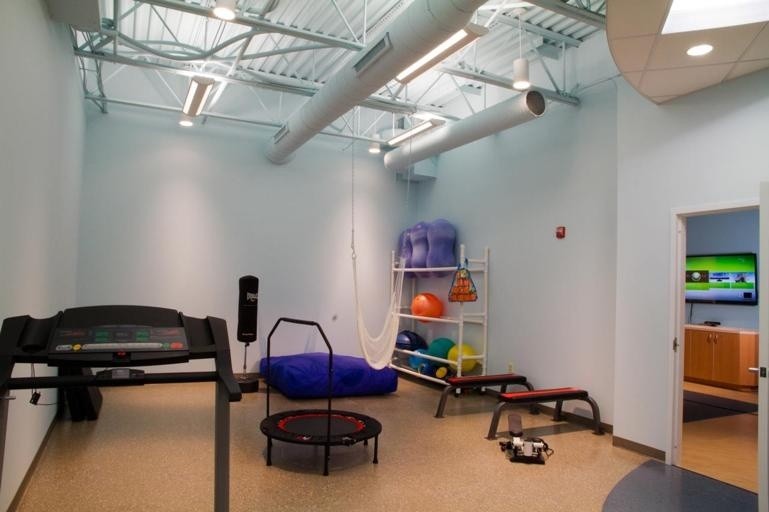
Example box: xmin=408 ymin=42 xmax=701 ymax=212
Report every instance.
xmin=435 ymin=373 xmax=604 ymax=440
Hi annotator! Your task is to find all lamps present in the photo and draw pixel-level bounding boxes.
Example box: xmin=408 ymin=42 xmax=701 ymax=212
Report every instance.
xmin=212 ymin=0 xmax=238 ymax=21
xmin=510 ymin=0 xmax=530 ymax=91
xmin=178 ymin=76 xmax=215 ymax=128
xmin=368 ymin=117 xmax=446 ymax=155
xmin=393 ymin=23 xmax=489 ymax=86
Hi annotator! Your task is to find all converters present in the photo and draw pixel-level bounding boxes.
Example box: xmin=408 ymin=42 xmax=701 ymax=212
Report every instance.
xmin=30 ymin=392 xmax=40 ymax=405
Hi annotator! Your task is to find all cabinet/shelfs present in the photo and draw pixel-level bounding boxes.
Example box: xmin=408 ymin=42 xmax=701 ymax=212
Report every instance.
xmin=388 ymin=244 xmax=491 ymax=397
xmin=685 ymin=324 xmax=760 ymax=392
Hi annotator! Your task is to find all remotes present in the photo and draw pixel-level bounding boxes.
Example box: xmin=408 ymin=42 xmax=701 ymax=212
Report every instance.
xmin=704 ymin=321 xmax=721 ymax=325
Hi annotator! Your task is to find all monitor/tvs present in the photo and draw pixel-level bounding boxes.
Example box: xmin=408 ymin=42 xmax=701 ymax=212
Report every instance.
xmin=685 ymin=252 xmax=758 ymax=306
